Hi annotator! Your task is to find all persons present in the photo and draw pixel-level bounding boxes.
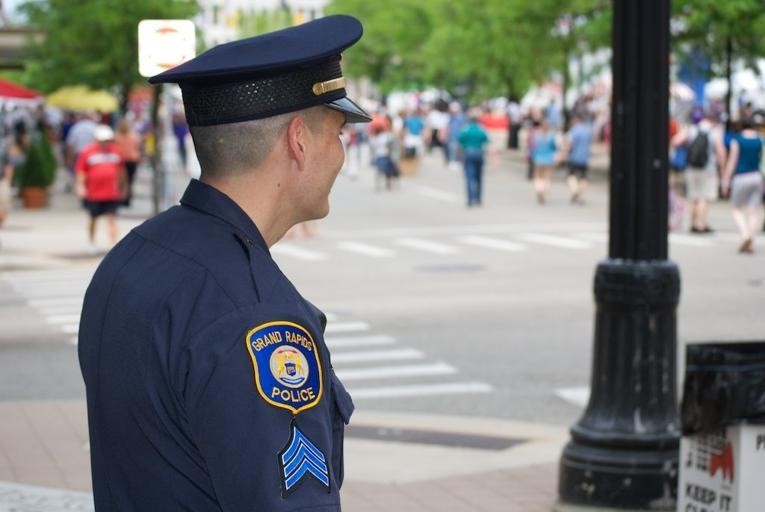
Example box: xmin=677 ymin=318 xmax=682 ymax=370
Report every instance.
xmin=341 ymin=88 xmax=612 ymax=209
xmin=669 ymin=77 xmax=765 ymax=254
xmin=1 ymin=87 xmax=201 ymax=244
xmin=78 ymin=14 xmax=374 ymax=512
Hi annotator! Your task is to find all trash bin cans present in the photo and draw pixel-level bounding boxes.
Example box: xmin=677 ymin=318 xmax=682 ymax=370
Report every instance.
xmin=677 ymin=341 xmax=765 ymax=512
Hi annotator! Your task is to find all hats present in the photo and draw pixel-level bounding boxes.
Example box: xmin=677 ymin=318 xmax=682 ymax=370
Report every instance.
xmin=147 ymin=14 xmax=373 ymax=127
xmin=94 ymin=125 xmax=115 ymax=144
